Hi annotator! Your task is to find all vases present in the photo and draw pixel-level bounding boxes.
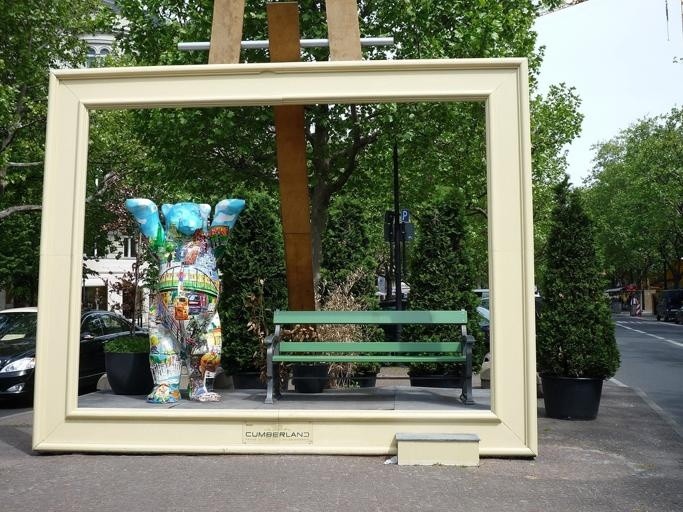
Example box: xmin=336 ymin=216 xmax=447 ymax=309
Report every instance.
xmin=230 ymin=371 xmax=261 ymax=389
xmin=352 ymin=372 xmax=376 ymax=388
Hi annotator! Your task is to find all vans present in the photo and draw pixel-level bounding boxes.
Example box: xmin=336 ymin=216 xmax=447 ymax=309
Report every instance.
xmin=656 ymin=288 xmax=682 ymax=322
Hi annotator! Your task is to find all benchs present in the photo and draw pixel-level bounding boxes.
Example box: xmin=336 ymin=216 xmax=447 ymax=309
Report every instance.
xmin=262 ymin=308 xmax=482 ymax=409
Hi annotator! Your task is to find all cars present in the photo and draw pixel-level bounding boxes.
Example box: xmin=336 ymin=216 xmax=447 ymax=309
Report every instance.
xmin=0 ymin=308 xmax=147 ymax=411
xmin=372 ymin=288 xmax=490 ymax=367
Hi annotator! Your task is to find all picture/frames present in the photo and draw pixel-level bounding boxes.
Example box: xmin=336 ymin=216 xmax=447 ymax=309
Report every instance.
xmin=29 ymin=59 xmax=545 ymax=461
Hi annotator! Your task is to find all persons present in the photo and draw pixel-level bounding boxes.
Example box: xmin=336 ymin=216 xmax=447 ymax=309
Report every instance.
xmin=166 ymin=252 xmax=173 ymax=266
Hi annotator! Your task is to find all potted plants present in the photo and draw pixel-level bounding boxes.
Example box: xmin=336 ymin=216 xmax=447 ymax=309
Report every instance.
xmin=284 ymin=324 xmax=328 ymax=394
xmin=402 ymin=184 xmax=471 ymax=387
xmin=544 ymin=167 xmax=619 ymax=420
xmin=100 ymin=335 xmax=153 ymax=396
